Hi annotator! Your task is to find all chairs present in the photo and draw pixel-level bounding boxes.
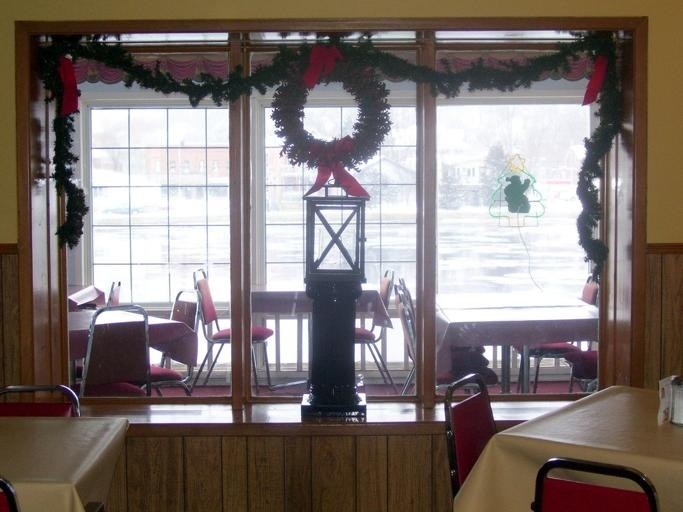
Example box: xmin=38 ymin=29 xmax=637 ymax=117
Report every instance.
xmin=392 ymin=278 xmax=498 ymax=392
xmin=512 ymin=274 xmax=599 ymax=393
xmin=565 ymin=348 xmax=598 ymax=393
xmin=350 ymin=269 xmax=396 ymax=386
xmin=442 ymin=372 xmax=661 ymax=512
xmin=81 ymin=280 xmax=122 ymax=309
xmin=187 ymin=268 xmax=274 ymax=392
xmin=78 ymin=303 xmax=152 ymax=392
xmin=151 ymin=288 xmax=202 ymax=392
xmin=0 ymin=385 xmax=80 ymax=417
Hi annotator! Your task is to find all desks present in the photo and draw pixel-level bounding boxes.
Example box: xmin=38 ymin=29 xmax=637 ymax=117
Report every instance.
xmin=0 ymin=416 xmax=131 ymax=512
xmin=425 ymin=285 xmax=599 ymax=385
xmin=452 ymin=385 xmax=683 ymax=512
xmin=243 ymin=286 xmax=392 ymax=379
xmin=69 ymin=306 xmax=193 ymax=373
